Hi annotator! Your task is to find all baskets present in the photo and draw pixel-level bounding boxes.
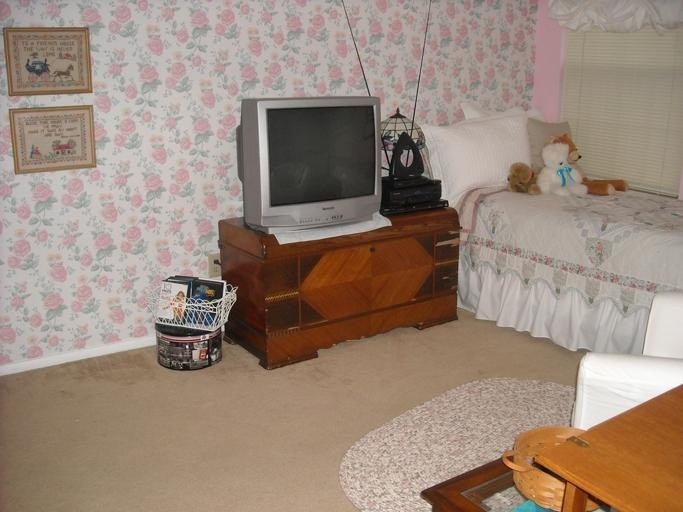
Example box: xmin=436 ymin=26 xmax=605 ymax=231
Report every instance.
xmin=503 ymin=428 xmax=602 ymax=512
xmin=149 ymin=284 xmax=240 ymax=333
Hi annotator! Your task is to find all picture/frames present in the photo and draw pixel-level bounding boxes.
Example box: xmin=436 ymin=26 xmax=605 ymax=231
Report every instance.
xmin=3 ymin=26 xmax=97 ymax=175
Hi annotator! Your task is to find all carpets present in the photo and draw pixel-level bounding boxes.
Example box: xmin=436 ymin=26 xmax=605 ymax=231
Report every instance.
xmin=337 ymin=377 xmax=576 ymax=512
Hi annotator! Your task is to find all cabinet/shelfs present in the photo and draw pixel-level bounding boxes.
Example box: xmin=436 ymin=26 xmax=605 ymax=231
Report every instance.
xmin=217 ymin=207 xmax=462 ymax=370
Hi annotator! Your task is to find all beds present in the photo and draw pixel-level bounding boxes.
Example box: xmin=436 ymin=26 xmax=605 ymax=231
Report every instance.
xmin=420 ymin=109 xmax=683 ymax=353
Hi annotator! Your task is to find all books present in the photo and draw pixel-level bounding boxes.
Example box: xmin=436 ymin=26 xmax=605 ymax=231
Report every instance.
xmin=156 ymin=275 xmax=226 ymax=326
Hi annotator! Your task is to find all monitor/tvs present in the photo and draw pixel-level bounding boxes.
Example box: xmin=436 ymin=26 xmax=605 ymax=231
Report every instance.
xmin=236 ymin=96 xmax=383 ymax=235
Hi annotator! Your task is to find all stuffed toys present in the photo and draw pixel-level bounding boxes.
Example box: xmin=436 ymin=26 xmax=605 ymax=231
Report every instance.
xmin=553 ymin=135 xmax=628 ymax=197
xmin=505 ymin=162 xmax=540 ymax=195
xmin=537 ymin=143 xmax=584 ymax=196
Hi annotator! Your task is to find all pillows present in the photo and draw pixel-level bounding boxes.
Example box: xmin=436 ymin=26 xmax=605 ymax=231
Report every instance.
xmin=421 ymin=105 xmax=572 ymax=209
